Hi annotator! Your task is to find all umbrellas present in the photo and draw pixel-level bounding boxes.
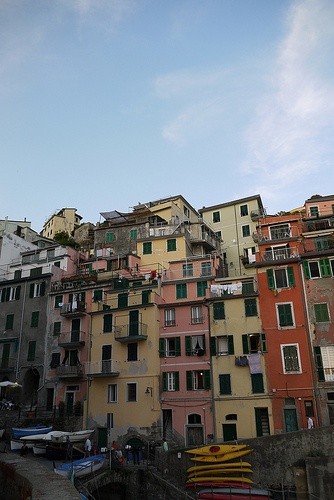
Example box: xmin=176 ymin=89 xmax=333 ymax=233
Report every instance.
xmin=0 ymin=381 xmax=22 ymax=388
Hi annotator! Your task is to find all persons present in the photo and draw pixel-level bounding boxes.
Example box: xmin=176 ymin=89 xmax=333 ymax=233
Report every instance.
xmin=84 ymin=437 xmax=98 ymax=455
xmin=0 ymin=399 xmax=13 ymax=411
xmin=162 ymin=439 xmax=168 ymax=452
xmin=112 ymin=441 xmax=119 ymax=451
xmin=305 ymin=414 xmax=313 ymax=429
xmin=1 ymin=430 xmax=11 ymax=453
xmin=20 ymin=441 xmax=28 ymax=456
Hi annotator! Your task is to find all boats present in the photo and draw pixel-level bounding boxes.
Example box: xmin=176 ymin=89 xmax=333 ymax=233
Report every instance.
xmin=19 ymin=430 xmax=95 ymax=456
xmin=10 ymin=425 xmax=55 ymax=440
xmin=185 ymin=443 xmax=272 ymax=500
xmin=54 ymin=454 xmax=104 ymax=478
